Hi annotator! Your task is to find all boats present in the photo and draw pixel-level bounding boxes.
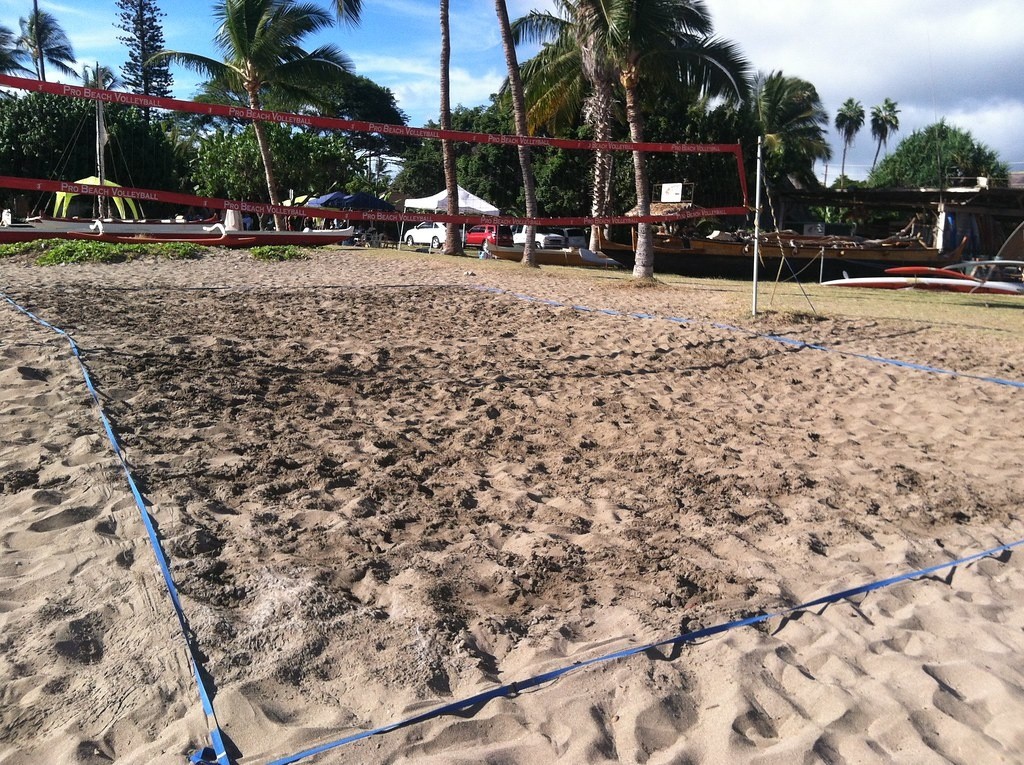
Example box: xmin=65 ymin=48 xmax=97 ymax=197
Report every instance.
xmin=0 ymin=61 xmax=354 ymax=247
xmin=599 ymin=221 xmax=1024 ymax=295
xmin=482 ymin=240 xmax=623 ymax=268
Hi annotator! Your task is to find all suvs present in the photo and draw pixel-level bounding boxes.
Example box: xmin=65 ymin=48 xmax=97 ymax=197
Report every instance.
xmin=466 ymin=225 xmax=514 ymax=251
xmin=513 ymin=224 xmax=587 ymax=250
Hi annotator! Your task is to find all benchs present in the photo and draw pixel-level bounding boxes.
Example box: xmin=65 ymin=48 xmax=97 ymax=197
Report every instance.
xmin=382 ymin=242 xmax=399 ymax=249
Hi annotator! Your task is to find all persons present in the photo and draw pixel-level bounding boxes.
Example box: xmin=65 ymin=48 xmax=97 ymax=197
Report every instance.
xmin=203 ymin=212 xmax=275 ymax=231
xmin=287 ymin=214 xmax=347 ymax=232
xmin=482 ymin=232 xmax=500 ymax=259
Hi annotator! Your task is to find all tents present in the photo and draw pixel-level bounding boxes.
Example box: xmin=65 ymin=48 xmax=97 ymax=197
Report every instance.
xmin=398 ymin=184 xmax=499 ymax=254
xmin=52 ymin=175 xmax=145 ymax=220
xmin=277 ymin=190 xmax=395 ymax=212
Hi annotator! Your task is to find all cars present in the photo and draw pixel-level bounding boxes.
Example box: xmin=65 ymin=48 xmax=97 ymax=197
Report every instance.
xmin=404 ymin=221 xmax=467 ymax=249
